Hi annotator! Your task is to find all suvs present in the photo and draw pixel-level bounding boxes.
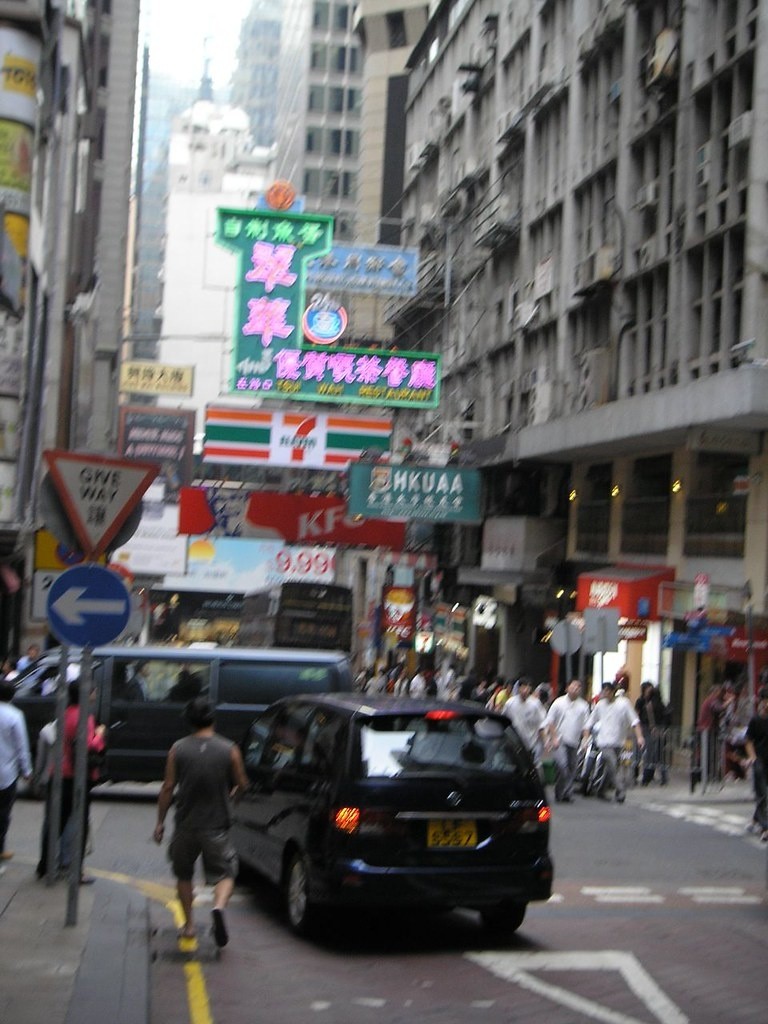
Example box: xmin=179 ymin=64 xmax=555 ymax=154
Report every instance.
xmin=224 ymin=693 xmax=554 ymax=950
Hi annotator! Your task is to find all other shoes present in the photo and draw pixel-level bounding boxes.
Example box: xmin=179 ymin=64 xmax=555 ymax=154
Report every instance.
xmin=616 ymin=791 xmax=626 ymax=802
xmin=211 ymin=908 xmax=229 ymax=947
xmin=2 ymin=850 xmax=14 ymax=858
xmin=81 ymin=875 xmax=95 ymax=882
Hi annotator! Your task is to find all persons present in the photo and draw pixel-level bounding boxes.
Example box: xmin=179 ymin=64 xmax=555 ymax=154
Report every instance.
xmin=357 ymin=662 xmax=768 ymax=842
xmin=0 ymin=646 xmax=152 ymax=885
xmin=154 ymin=697 xmax=254 ymax=949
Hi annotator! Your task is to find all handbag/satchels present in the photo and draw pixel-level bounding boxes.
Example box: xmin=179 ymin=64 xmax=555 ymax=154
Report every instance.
xmin=72 ymin=746 xmax=110 ymax=788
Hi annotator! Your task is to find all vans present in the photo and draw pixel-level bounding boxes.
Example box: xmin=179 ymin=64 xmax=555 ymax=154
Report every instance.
xmin=6 ymin=648 xmax=351 ymax=800
